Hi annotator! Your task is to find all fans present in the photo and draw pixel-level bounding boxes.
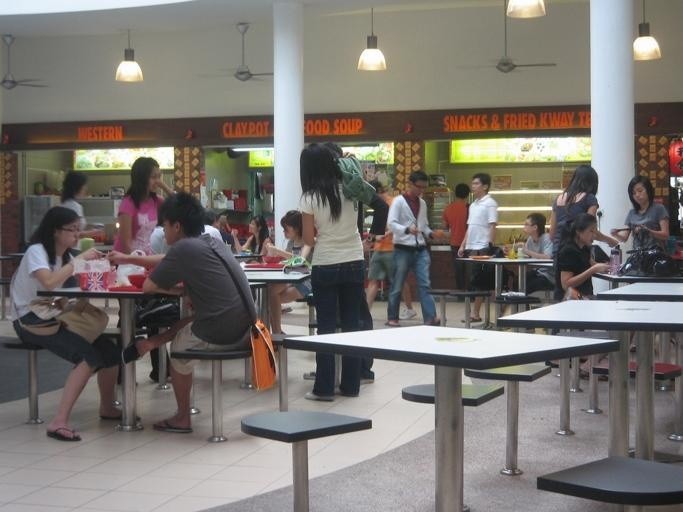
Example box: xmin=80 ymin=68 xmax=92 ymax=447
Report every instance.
xmin=0 ymin=35 xmax=50 ymax=90
xmin=216 ymin=22 xmax=274 ymax=82
xmin=470 ymin=0 xmax=557 ymax=73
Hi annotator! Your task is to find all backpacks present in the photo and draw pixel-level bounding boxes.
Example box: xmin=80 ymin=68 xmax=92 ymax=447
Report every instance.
xmin=474 ymin=242 xmax=508 ymax=289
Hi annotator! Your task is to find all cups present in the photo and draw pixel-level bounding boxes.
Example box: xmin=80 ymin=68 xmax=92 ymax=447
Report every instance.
xmin=506 ymin=245 xmax=516 ymax=259
xmin=433 ymin=229 xmax=443 ymax=238
xmin=225 ymin=244 xmax=231 ymax=255
xmin=516 ymin=245 xmax=525 ymax=258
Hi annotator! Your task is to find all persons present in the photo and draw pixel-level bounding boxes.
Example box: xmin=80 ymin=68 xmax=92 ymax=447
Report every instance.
xmin=9 ymin=158 xmax=257 ymax=439
xmin=367 ymin=170 xmax=499 ymax=321
xmin=266 ymin=141 xmax=375 ymax=401
xmin=503 ymin=213 xmax=555 ymax=292
xmin=549 ymin=164 xmax=670 ymax=303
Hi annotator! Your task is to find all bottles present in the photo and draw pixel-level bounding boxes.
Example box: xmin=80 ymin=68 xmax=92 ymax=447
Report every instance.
xmin=616 ymin=245 xmax=623 ymax=264
xmin=609 ymin=250 xmax=619 ymax=274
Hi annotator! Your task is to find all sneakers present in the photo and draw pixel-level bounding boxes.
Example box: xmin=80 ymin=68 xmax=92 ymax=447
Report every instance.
xmin=386 ymin=309 xmax=440 ymax=327
xmin=461 ymin=317 xmax=481 ymax=322
xmin=304 ymin=373 xmax=374 ymax=401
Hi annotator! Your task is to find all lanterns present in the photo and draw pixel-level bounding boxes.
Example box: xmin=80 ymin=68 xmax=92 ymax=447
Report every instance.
xmin=667 ymin=137 xmax=683 ymax=177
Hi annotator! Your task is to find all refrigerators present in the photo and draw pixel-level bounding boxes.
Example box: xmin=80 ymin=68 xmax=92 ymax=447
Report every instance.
xmin=24 ymin=195 xmax=60 ymax=249
xmin=229 ymin=171 xmax=264 ymax=226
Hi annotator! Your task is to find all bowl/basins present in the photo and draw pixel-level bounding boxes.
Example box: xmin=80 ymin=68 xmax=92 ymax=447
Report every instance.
xmin=262 ymin=255 xmax=281 ymax=263
xmin=127 ymin=274 xmax=145 ymax=287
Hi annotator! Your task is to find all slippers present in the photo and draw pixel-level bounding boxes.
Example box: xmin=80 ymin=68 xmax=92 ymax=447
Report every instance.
xmin=101 ymin=410 xmax=140 ymax=420
xmin=153 ymin=420 xmax=192 ymax=433
xmin=122 ymin=336 xmax=145 ymax=366
xmin=48 ymin=428 xmax=81 ymax=441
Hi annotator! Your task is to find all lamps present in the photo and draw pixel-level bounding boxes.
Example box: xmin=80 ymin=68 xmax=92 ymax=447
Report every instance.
xmin=632 ymin=0 xmax=662 ymax=61
xmin=116 ymin=29 xmax=144 ymax=82
xmin=358 ymin=7 xmax=387 ymax=71
xmin=506 ymin=0 xmax=547 ymax=18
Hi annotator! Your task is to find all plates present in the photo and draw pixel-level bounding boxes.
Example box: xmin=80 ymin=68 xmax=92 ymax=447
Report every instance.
xmin=469 ymin=255 xmax=492 ymax=259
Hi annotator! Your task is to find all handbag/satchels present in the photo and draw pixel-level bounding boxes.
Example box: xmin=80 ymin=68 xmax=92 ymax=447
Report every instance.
xmin=31 ymin=297 xmax=108 ymax=344
xmin=250 ymin=319 xmax=277 ymax=391
xmin=619 ymin=245 xmax=679 ymax=276
